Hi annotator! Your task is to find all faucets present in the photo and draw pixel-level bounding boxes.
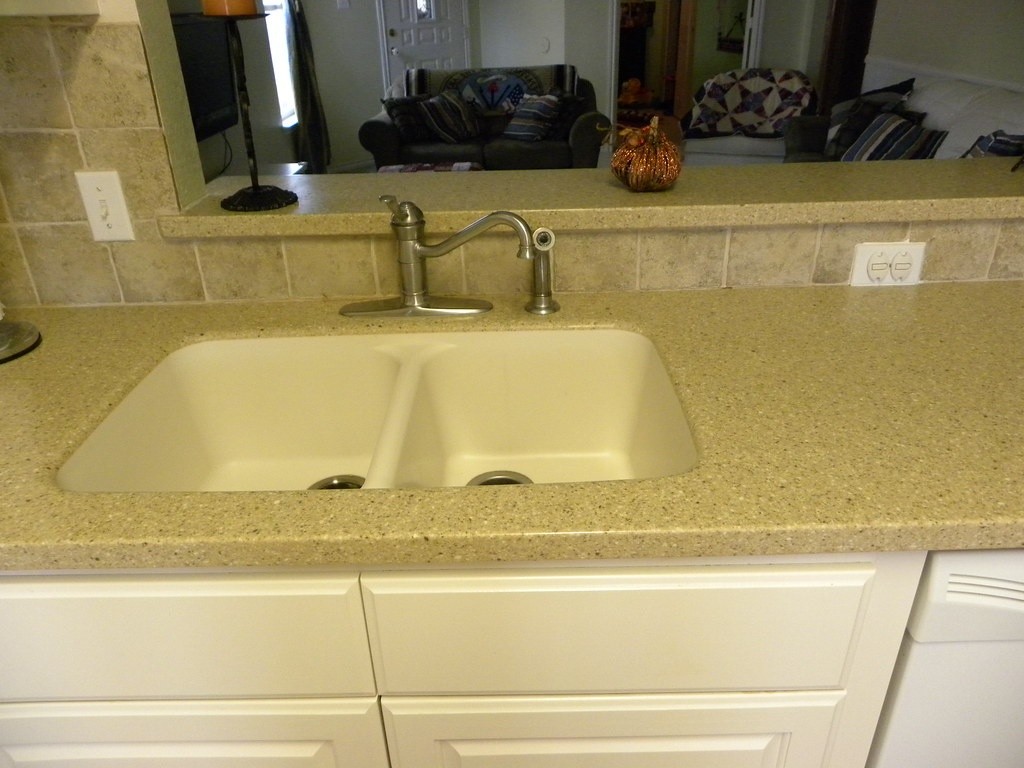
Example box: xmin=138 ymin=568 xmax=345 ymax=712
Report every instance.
xmin=379 ymin=192 xmax=536 ymax=318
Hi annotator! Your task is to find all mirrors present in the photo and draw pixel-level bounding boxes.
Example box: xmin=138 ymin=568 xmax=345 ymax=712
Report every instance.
xmin=137 ymin=0 xmax=1024 ymax=183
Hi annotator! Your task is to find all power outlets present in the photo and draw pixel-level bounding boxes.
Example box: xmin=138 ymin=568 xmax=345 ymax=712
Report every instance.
xmin=851 ymin=242 xmax=926 ymax=286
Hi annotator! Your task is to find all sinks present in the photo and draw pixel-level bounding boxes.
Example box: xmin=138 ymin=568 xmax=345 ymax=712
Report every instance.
xmin=54 ymin=322 xmax=426 ymax=495
xmin=364 ymin=323 xmax=705 ymax=489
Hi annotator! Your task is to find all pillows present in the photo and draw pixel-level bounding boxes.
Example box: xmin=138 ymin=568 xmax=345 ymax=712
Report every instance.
xmin=838 ymin=113 xmax=949 ymax=159
xmin=379 ymin=93 xmax=433 ymax=141
xmin=824 ymin=76 xmax=915 ymax=158
xmin=961 ymin=129 xmax=1024 ymax=157
xmin=422 ymin=89 xmax=482 ymax=143
xmin=503 ymin=90 xmax=561 ymax=140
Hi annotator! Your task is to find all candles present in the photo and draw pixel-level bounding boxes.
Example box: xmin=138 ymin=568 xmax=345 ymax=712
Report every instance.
xmin=203 ymin=0 xmax=257 ymax=16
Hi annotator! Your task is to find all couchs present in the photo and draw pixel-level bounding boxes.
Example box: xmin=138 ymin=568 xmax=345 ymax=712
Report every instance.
xmin=683 ymin=68 xmax=818 ymax=156
xmin=778 ymin=109 xmax=838 ymax=162
xmin=357 ymin=62 xmax=611 ymax=169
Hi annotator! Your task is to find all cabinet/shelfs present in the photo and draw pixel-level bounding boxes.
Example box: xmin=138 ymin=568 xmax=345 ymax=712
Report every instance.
xmin=0 ymin=552 xmax=928 ymax=768
